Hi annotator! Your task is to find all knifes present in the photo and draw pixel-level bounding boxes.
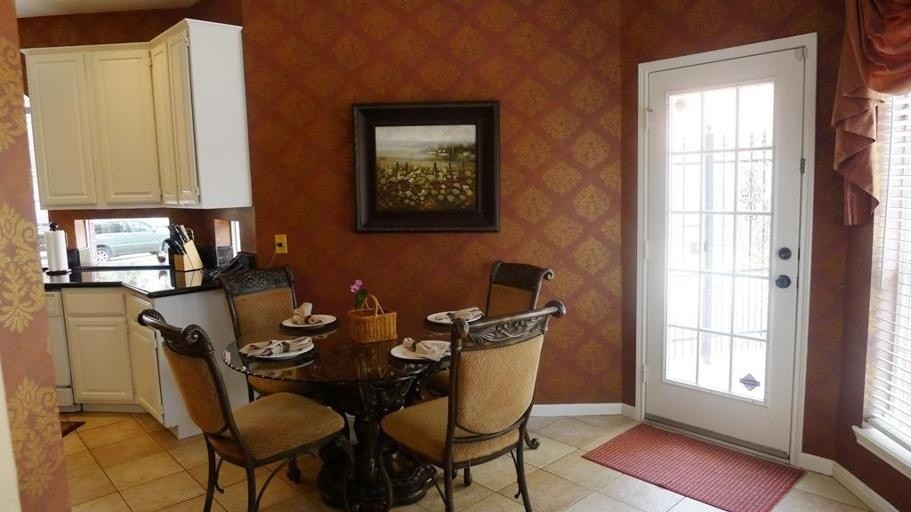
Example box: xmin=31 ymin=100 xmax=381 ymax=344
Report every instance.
xmin=164 ymin=223 xmax=185 ymax=256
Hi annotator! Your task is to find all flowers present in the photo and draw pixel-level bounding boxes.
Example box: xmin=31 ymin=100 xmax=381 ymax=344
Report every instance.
xmin=349 ymin=279 xmax=369 ymax=307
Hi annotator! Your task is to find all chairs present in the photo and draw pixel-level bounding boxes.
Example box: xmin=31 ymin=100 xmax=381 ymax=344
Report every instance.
xmin=136 ymin=309 xmax=351 ymax=512
xmin=221 ymin=267 xmax=352 ymax=484
xmin=483 ymin=259 xmax=555 ymax=449
xmin=381 ymin=299 xmax=566 ymax=512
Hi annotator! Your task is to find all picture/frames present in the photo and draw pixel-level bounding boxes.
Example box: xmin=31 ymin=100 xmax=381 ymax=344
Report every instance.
xmin=350 ymin=100 xmax=501 ymax=233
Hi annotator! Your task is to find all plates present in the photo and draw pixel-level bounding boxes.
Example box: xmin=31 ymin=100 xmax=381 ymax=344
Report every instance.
xmin=280 ymin=314 xmax=336 ymax=331
xmin=425 ymin=310 xmax=482 ymax=325
xmin=388 ymin=340 xmax=448 ymax=360
xmin=255 ymin=340 xmax=314 ymax=364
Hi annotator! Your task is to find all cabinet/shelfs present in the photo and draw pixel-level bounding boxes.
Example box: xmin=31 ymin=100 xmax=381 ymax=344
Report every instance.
xmin=26 ymin=50 xmax=161 ymax=210
xmin=124 ymin=294 xmax=249 ymax=439
xmin=43 ymin=291 xmax=74 ymax=406
xmin=61 ymin=288 xmax=134 ymax=403
xmin=149 ymin=18 xmax=253 ymax=210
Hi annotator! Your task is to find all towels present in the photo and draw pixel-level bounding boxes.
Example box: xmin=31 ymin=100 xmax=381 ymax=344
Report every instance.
xmin=250 ymin=335 xmax=312 ymax=355
xmin=294 ymin=301 xmax=313 ymax=324
xmin=449 ymin=305 xmax=484 ymax=323
xmin=403 ymin=335 xmax=451 ymax=361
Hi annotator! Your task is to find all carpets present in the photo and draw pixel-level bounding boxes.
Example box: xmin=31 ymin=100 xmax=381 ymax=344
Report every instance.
xmin=580 ymin=421 xmax=810 ymax=512
xmin=61 ymin=421 xmax=87 ymax=438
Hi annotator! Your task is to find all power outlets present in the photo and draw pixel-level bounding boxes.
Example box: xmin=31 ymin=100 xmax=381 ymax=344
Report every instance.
xmin=274 ymin=234 xmax=288 ymax=253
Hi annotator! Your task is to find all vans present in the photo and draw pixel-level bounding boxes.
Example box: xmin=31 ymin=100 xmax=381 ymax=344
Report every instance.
xmin=94 ymin=219 xmax=170 ymax=262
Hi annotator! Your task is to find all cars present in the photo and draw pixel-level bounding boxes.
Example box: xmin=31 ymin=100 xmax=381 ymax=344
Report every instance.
xmin=37 ymin=220 xmax=50 ymax=251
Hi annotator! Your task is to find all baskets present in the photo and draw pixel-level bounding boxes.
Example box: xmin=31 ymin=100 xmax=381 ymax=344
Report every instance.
xmin=345 ymin=294 xmax=398 ymax=345
